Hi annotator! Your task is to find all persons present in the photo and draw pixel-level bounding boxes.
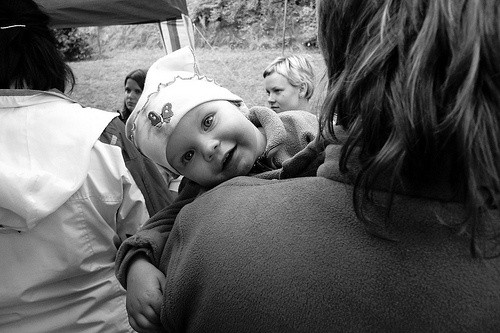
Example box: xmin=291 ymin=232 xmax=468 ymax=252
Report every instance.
xmin=115 ymin=46 xmax=335 ymax=333
xmin=0 ymin=0 xmax=151 ymax=333
xmin=152 ymin=0 xmax=500 ymax=333
xmin=116 ymin=69 xmax=147 ymax=124
xmin=263 ymin=56 xmax=315 ymax=114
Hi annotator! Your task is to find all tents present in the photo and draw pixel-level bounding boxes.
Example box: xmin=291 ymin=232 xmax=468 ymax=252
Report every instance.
xmin=35 ymin=0 xmax=195 ymax=54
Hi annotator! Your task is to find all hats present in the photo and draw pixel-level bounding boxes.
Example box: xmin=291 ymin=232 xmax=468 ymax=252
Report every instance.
xmin=122 ymin=45 xmax=244 ymax=173
